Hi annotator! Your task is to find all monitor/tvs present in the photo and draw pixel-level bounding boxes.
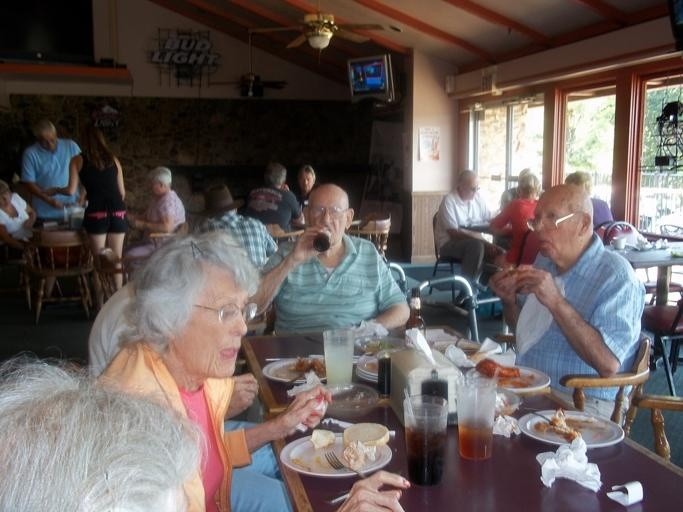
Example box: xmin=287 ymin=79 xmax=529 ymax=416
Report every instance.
xmin=0 ymin=0 xmax=97 ymax=67
xmin=346 ymin=53 xmax=397 ymax=106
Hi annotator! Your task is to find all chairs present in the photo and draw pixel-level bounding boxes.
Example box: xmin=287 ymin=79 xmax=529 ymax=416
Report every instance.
xmin=95 ymin=248 xmax=138 ymax=302
xmin=149 ymin=222 xmax=188 ymax=251
xmin=496 ymin=331 xmax=650 ymax=423
xmin=594 ymin=221 xmax=614 ymax=243
xmin=22 ymin=241 xmax=92 ymax=326
xmin=348 ymin=213 xmax=391 ymax=262
xmin=640 ymin=292 xmax=683 ymax=398
xmin=265 ymin=225 xmax=304 ymax=246
xmin=428 ymin=213 xmax=464 ymax=302
xmin=661 ymin=225 xmax=683 ymax=236
xmin=604 ymin=221 xmax=639 ymax=246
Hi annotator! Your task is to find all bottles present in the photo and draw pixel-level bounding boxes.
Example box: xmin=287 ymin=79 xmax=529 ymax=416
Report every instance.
xmin=313 ymin=230 xmax=330 ymax=252
xmin=406 ymin=289 xmax=426 ymax=337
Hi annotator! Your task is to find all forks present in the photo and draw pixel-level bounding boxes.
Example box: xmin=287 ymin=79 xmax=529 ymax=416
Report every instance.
xmin=324 ymin=451 xmax=364 ymax=481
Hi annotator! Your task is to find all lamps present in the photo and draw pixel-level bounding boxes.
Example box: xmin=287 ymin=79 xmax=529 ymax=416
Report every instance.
xmin=306 ymin=32 xmax=332 ymax=51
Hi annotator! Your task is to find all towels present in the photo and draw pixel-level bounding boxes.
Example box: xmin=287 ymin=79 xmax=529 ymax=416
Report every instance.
xmin=516 ymin=279 xmax=565 ymax=355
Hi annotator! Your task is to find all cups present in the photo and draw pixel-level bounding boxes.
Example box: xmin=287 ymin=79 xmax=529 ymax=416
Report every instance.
xmin=403 ymin=396 xmax=448 ymax=488
xmin=455 ymin=378 xmax=497 ymax=462
xmin=609 ymin=235 xmax=627 ymax=251
xmin=322 ymin=330 xmax=354 ymax=388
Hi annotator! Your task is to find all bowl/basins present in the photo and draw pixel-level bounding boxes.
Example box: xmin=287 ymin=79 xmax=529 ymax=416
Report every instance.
xmin=327 ymin=385 xmax=379 ymax=418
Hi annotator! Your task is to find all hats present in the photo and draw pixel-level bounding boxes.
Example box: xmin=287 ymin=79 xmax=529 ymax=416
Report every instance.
xmin=202 ymin=185 xmax=245 ymax=214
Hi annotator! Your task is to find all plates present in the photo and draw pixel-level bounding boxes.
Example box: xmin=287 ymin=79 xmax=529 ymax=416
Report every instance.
xmin=280 ymin=434 xmax=393 ymax=478
xmin=262 ymin=357 xmax=326 ymax=383
xmin=466 ymin=366 xmax=551 ymax=393
xmin=355 ymin=337 xmax=404 ymax=352
xmin=519 ymin=410 xmax=625 ymax=448
xmin=356 ymin=356 xmax=378 ymax=382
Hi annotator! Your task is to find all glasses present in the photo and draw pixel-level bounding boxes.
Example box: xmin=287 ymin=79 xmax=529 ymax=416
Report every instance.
xmin=527 ymin=211 xmax=578 ymax=232
xmin=308 ymin=206 xmax=348 ymax=217
xmin=194 ymin=303 xmax=256 ymax=324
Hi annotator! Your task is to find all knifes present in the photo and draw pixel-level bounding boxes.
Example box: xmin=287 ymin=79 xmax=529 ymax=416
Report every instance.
xmin=324 ymin=470 xmax=407 ymax=506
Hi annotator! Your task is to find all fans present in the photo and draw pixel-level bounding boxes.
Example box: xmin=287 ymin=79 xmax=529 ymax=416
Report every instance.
xmin=249 ymin=0 xmax=402 ymax=48
xmin=209 ymin=34 xmax=286 ymax=96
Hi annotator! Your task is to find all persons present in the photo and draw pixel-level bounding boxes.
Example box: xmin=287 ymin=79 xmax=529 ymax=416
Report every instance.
xmin=93 ymin=227 xmax=410 ymax=511
xmin=245 ymin=163 xmax=308 ymax=242
xmin=253 ymin=184 xmax=411 ymax=335
xmin=491 ymin=184 xmax=645 ymax=422
xmin=121 ymin=166 xmax=185 ymax=281
xmin=46 ymin=124 xmax=128 ymax=317
xmin=195 ymin=183 xmax=280 ymax=269
xmin=293 ymin=164 xmax=319 ymax=203
xmin=435 ymin=171 xmax=496 ymax=309
xmin=491 ymin=171 xmax=540 ymax=267
xmin=21 ymin=120 xmax=88 ymax=219
xmin=86 ymin=215 xmax=298 ymax=507
xmin=564 ymin=172 xmax=614 ymax=231
xmin=0 ymin=354 xmax=205 ymax=512
xmin=499 ymin=168 xmax=533 ymax=212
xmin=0 ymin=179 xmax=40 ymax=250
